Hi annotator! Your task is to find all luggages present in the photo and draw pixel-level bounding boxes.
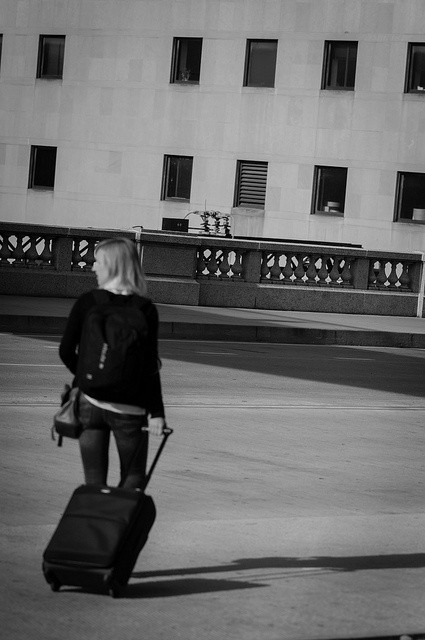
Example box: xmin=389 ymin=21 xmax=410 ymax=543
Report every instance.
xmin=43 ymin=425 xmax=175 ymax=597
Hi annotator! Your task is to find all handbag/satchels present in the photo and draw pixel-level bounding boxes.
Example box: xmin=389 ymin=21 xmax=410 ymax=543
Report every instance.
xmin=50 ymin=383 xmax=83 ymax=447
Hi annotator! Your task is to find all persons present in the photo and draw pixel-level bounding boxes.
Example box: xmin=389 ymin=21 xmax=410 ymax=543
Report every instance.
xmin=58 ymin=236 xmax=166 ymax=488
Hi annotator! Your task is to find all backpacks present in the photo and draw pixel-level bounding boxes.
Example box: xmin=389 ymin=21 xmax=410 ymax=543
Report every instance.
xmin=77 ymin=289 xmax=153 ymax=397
